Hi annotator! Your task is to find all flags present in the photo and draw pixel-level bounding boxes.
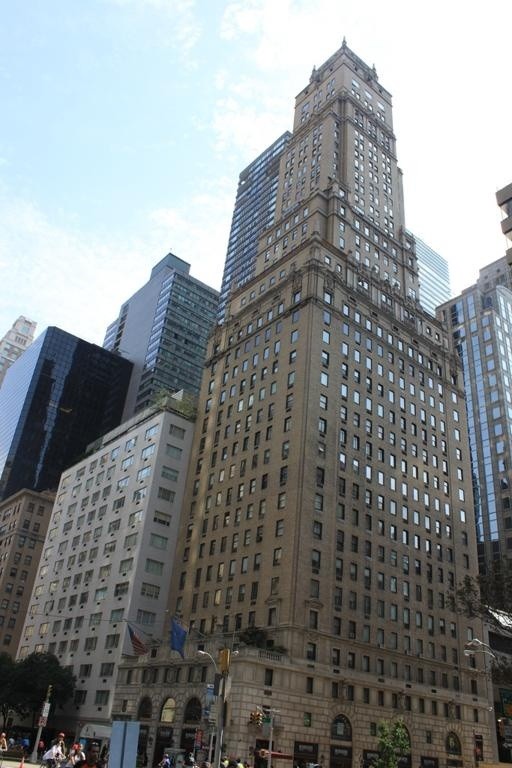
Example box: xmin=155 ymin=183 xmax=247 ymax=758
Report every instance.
xmin=170 ymin=616 xmax=188 ymax=660
xmin=128 ymin=621 xmax=149 ymax=656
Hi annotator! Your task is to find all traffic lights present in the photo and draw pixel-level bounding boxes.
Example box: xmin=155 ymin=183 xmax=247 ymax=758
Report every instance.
xmin=249 ymin=712 xmax=263 ymax=729
xmin=497 ymin=718 xmax=509 ymax=737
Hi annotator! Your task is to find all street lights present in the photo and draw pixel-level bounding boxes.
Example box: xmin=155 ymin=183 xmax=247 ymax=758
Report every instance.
xmin=464 ymin=639 xmax=510 ymax=763
xmin=195 ymin=650 xmax=239 ymax=768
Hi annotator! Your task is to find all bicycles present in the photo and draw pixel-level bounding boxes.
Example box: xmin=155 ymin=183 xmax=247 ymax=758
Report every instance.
xmin=39 ymin=757 xmax=66 ymax=768
xmin=0 ymin=749 xmax=7 ymax=768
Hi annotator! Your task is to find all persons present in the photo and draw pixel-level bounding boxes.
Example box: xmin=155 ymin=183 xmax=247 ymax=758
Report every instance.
xmin=1 ymin=732 xmax=111 ymax=768
xmin=157 ymin=751 xmax=250 ymax=768
